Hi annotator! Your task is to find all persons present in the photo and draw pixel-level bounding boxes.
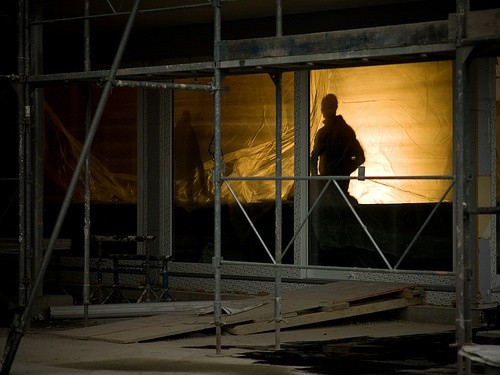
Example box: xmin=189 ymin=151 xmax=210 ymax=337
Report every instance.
xmin=310 ymin=92 xmax=366 ymax=196
